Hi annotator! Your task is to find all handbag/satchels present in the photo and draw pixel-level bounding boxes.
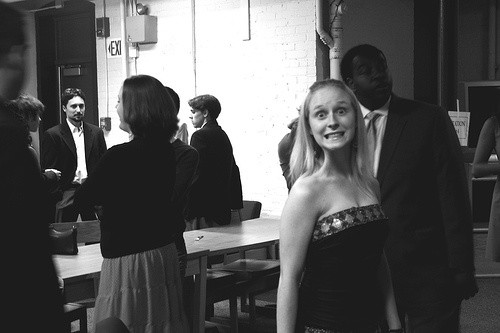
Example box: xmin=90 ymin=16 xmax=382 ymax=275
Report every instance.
xmin=49 ymin=224 xmax=78 ymax=255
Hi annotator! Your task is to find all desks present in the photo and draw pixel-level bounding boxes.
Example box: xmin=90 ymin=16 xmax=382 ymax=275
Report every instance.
xmin=460 ymin=147 xmax=500 ymax=278
xmin=52 ymin=242 xmax=210 ymax=333
xmin=183 ymin=216 xmax=280 ymax=333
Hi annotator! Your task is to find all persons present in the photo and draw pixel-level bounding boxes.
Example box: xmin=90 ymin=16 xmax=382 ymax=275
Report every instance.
xmin=470 ymin=102 xmax=500 ymax=263
xmin=0 ymin=1 xmax=244 ymax=333
xmin=339 ymin=43 xmax=479 ymax=332
xmin=275 ymin=79 xmax=402 ymax=332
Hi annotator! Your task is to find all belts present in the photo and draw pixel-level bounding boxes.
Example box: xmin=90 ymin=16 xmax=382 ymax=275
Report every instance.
xmin=70 ymin=184 xmax=81 ymax=188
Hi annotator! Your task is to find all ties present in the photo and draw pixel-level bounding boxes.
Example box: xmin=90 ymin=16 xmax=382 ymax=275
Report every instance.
xmin=361 ymin=112 xmax=381 ymax=177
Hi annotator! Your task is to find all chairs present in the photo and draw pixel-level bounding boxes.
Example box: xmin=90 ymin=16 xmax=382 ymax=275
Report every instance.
xmin=51 ymin=220 xmax=102 ymax=333
xmin=184 ymin=200 xmax=282 ymax=333
